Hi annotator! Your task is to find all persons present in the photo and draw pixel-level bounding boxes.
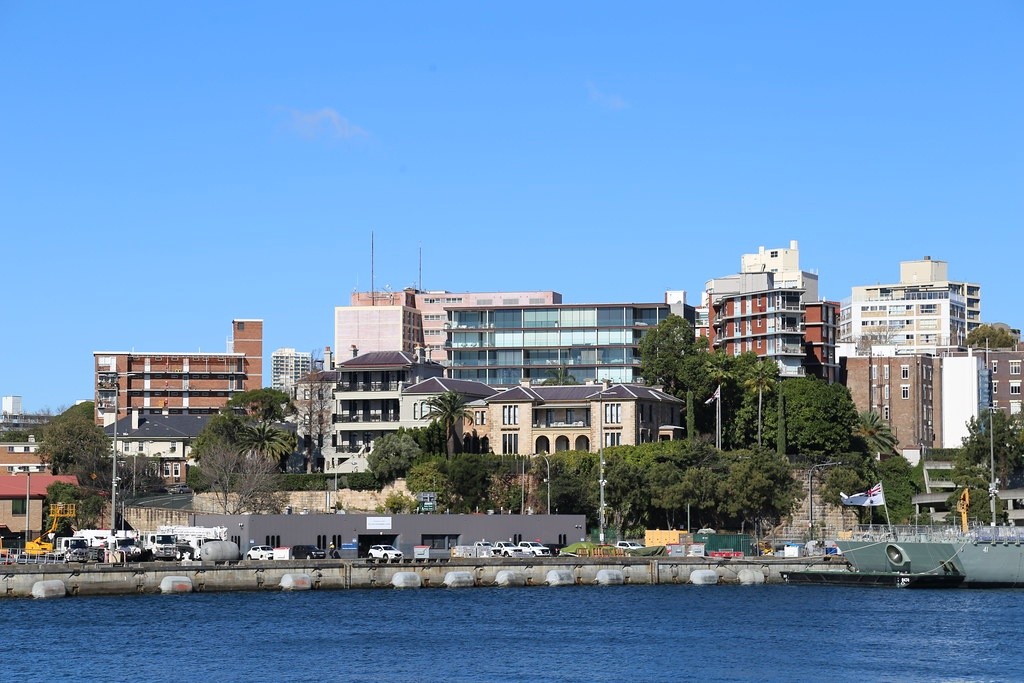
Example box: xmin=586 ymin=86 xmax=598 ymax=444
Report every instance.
xmin=329 ymin=541 xmax=335 ymax=559
xmin=554 ymin=548 xmax=561 ymax=556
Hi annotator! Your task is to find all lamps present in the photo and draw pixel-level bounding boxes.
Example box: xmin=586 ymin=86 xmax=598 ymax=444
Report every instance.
xmin=379 ymin=531 xmax=383 ymax=535
xmin=576 ymin=525 xmax=578 ymax=529
xmin=239 ymin=522 xmax=244 ymax=527
xmin=579 ymin=525 xmax=582 ymax=529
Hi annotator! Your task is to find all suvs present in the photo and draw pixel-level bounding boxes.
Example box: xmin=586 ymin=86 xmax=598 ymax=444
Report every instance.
xmin=369 ymin=545 xmax=404 ymax=559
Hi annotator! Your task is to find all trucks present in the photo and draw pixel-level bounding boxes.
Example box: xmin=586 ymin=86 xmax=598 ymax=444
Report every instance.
xmin=0 ymin=504 xmax=90 ymax=563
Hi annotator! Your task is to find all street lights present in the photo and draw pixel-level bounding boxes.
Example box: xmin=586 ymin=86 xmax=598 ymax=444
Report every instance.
xmin=112 ymin=380 xmax=118 ymax=550
xmin=334 ymin=462 xmax=357 ymax=514
xmin=809 ymin=461 xmax=844 ymax=542
xmin=599 ymin=392 xmax=618 ymax=543
xmin=536 ymin=454 xmax=550 ymax=516
xmin=989 ymin=407 xmax=1006 ymax=525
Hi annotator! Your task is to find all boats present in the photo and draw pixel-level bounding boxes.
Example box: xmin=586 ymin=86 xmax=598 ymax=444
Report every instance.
xmin=779 ymin=539 xmax=1024 ymax=587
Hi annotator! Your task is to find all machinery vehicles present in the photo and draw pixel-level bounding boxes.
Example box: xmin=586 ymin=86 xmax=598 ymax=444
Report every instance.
xmin=72 ymin=529 xmax=141 ymax=562
xmin=136 ymin=525 xmax=228 ymax=559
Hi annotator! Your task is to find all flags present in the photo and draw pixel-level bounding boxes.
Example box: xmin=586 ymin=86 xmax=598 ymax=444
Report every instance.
xmin=705 ymin=386 xmax=719 ymax=404
xmin=839 ymin=483 xmax=884 ymax=507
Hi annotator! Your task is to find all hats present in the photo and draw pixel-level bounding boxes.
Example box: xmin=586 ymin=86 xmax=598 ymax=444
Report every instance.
xmin=330 ymin=542 xmax=333 ymax=544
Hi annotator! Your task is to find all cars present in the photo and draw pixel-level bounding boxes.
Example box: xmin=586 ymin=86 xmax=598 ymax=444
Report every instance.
xmin=543 ymin=543 xmax=567 ymax=555
xmin=169 ymin=484 xmax=191 ymax=494
xmin=292 ymin=545 xmax=326 ymax=560
xmin=247 ymin=545 xmax=275 ymax=560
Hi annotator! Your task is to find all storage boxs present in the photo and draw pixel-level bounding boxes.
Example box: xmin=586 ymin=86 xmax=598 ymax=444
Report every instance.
xmin=693 ymin=534 xmax=753 ymax=556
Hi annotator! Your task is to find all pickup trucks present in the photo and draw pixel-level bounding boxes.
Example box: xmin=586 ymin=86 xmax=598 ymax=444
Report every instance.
xmin=517 ymin=541 xmax=551 ymax=558
xmin=614 ymin=540 xmax=645 ymax=550
xmin=454 ymin=540 xmax=501 ymax=558
xmin=492 ymin=542 xmax=523 ymax=558
